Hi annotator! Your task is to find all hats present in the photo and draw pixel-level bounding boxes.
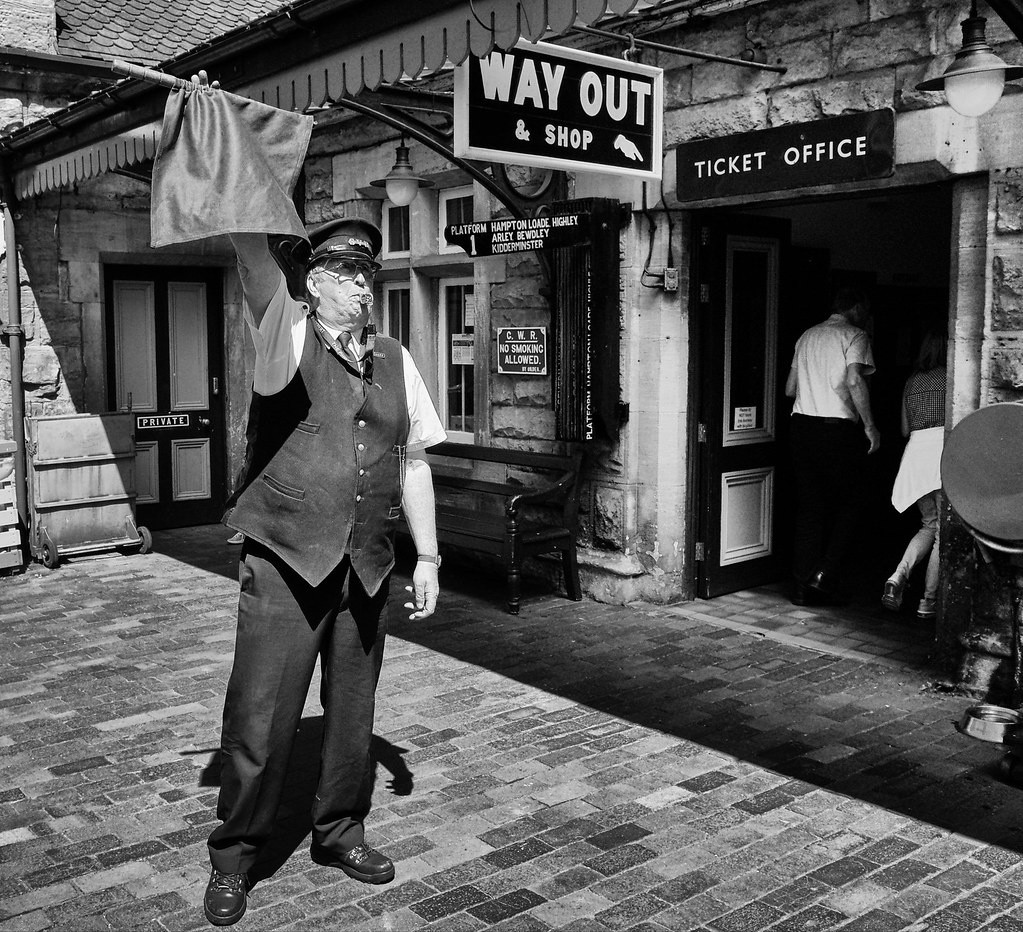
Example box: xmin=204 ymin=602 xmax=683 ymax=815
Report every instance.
xmin=289 ymin=216 xmax=383 ymax=272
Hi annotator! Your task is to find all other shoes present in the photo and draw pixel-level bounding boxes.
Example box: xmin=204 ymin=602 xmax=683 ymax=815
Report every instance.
xmin=881 ymin=581 xmax=903 ymax=613
xmin=917 ymin=596 xmax=937 ymax=619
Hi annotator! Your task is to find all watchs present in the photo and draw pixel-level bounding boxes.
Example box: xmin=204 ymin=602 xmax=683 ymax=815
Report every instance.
xmin=418 ymin=555 xmax=442 ymax=567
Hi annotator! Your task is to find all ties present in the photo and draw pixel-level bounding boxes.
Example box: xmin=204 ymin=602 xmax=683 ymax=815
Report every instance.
xmin=337 ymin=331 xmax=359 ymax=374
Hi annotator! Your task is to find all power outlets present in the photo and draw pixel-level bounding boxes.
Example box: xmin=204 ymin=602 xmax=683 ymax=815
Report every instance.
xmin=665 ymin=269 xmax=679 ymax=291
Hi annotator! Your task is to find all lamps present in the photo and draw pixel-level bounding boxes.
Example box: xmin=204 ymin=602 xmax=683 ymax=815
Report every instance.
xmin=913 ymin=0 xmax=1023 ymax=117
xmin=370 ymin=134 xmax=435 ymax=206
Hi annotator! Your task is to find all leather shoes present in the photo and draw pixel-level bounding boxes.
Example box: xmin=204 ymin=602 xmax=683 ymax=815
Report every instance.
xmin=204 ymin=867 xmax=251 ymax=926
xmin=790 ymin=584 xmax=814 ymax=607
xmin=808 ymin=568 xmax=828 ymax=596
xmin=325 ymin=841 xmax=395 ymax=885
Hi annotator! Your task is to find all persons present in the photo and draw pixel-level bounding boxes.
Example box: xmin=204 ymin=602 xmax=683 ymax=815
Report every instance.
xmin=784 ymin=283 xmax=882 ymax=602
xmin=880 ymin=323 xmax=947 ymax=618
xmin=190 ymin=69 xmax=447 ymax=926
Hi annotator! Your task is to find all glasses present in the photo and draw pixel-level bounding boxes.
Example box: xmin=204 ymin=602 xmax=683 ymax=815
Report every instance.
xmin=311 ymin=261 xmax=379 ymax=281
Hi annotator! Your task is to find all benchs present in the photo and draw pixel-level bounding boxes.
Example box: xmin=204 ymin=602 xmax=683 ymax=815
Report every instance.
xmin=426 ymin=442 xmax=582 ymax=615
